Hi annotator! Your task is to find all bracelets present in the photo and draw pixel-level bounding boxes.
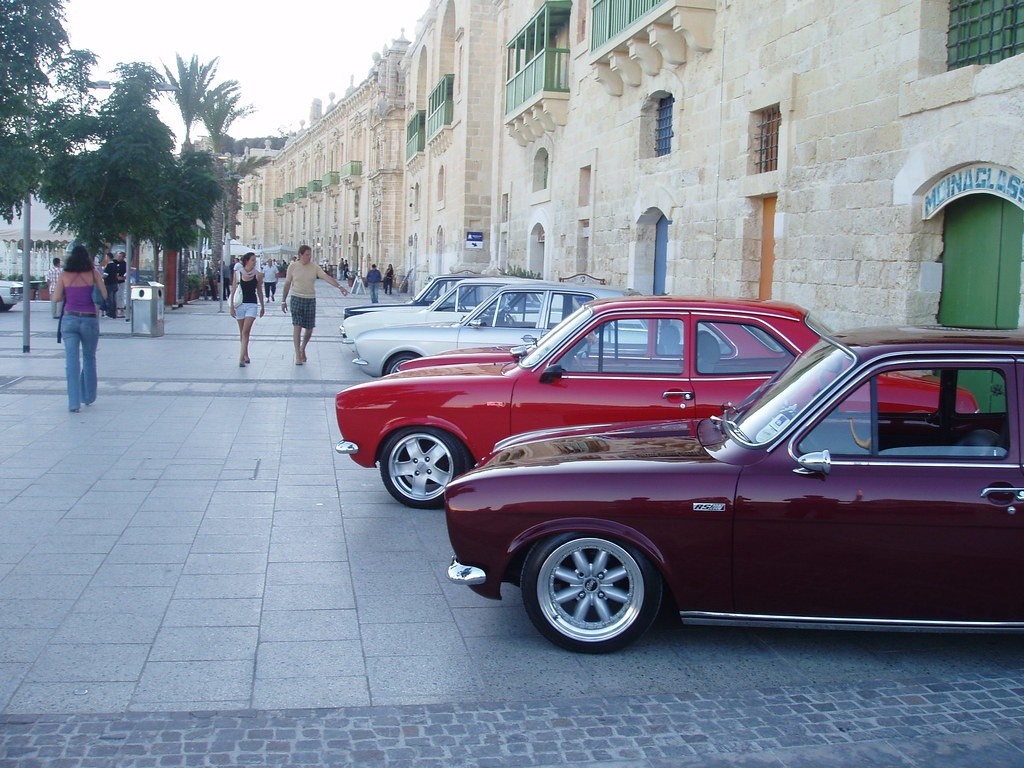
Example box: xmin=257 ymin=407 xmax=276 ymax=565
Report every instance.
xmin=282 ymin=301 xmax=286 ymax=304
xmin=338 ymin=285 xmax=341 ymax=288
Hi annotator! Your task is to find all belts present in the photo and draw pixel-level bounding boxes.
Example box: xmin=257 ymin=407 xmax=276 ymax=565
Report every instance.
xmin=64 ymin=311 xmax=96 ymax=317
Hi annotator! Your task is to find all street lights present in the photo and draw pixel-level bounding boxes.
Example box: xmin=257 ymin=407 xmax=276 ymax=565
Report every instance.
xmin=85 ymin=80 xmax=180 ymax=321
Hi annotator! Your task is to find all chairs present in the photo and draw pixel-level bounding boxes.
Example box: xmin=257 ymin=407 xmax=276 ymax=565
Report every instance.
xmin=697 ymin=330 xmax=719 ymax=373
xmin=657 ymin=326 xmax=680 ymax=355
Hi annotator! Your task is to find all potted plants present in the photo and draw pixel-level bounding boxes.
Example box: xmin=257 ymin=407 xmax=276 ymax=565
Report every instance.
xmin=188 ymin=274 xmax=200 ymax=301
xmin=17 ymin=275 xmax=23 ymax=293
xmin=39 ymin=277 xmax=49 ymax=300
xmin=8 ymin=273 xmax=17 ymax=293
xmin=30 ymin=276 xmax=37 ymax=300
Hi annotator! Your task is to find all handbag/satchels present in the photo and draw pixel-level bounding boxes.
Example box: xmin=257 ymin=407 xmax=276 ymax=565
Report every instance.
xmin=228 ymin=271 xmax=243 ymax=307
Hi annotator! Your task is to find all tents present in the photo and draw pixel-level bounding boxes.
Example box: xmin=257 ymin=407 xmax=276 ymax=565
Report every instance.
xmin=0 ymin=191 xmax=79 ymax=275
xmin=201 ymin=238 xmax=299 ymax=261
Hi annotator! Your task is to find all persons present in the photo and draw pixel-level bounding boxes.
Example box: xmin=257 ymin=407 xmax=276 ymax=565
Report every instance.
xmin=385 ymin=263 xmax=396 ymax=294
xmin=364 ymin=264 xmax=382 ymax=303
xmin=319 ymin=258 xmax=330 ymax=274
xmin=338 ymin=258 xmax=348 ymax=281
xmin=261 ymin=256 xmax=297 ymax=269
xmin=53 ymin=245 xmax=108 ymax=412
xmin=46 ymin=258 xmax=63 ymax=319
xmin=280 ymin=244 xmax=350 ymax=365
xmin=229 ymin=258 xmax=243 ymax=287
xmin=262 ymin=258 xmax=279 ymax=303
xmin=92 ymin=252 xmax=126 ymax=318
xmin=229 ymin=252 xmax=264 ymax=367
xmin=206 ymin=261 xmax=230 ymax=301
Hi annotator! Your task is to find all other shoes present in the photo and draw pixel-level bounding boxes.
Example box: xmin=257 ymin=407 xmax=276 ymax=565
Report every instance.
xmin=239 ymin=363 xmax=245 ymax=367
xmin=303 ymin=357 xmax=307 ymax=362
xmin=296 ymin=357 xmax=302 ymax=365
xmin=271 ymin=295 xmax=275 ymax=301
xmin=245 ymin=358 xmax=251 ymax=363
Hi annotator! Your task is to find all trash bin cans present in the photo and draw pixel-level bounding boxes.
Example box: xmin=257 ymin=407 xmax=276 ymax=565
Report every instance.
xmin=130 ymin=281 xmax=164 ymax=337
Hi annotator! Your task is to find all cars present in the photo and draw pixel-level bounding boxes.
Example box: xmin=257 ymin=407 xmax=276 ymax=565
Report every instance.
xmin=340 ymin=273 xmax=785 ymax=379
xmin=444 ymin=325 xmax=1024 ymax=655
xmin=0 ymin=279 xmax=23 ymax=312
xmin=336 ymin=296 xmax=977 ymax=509
